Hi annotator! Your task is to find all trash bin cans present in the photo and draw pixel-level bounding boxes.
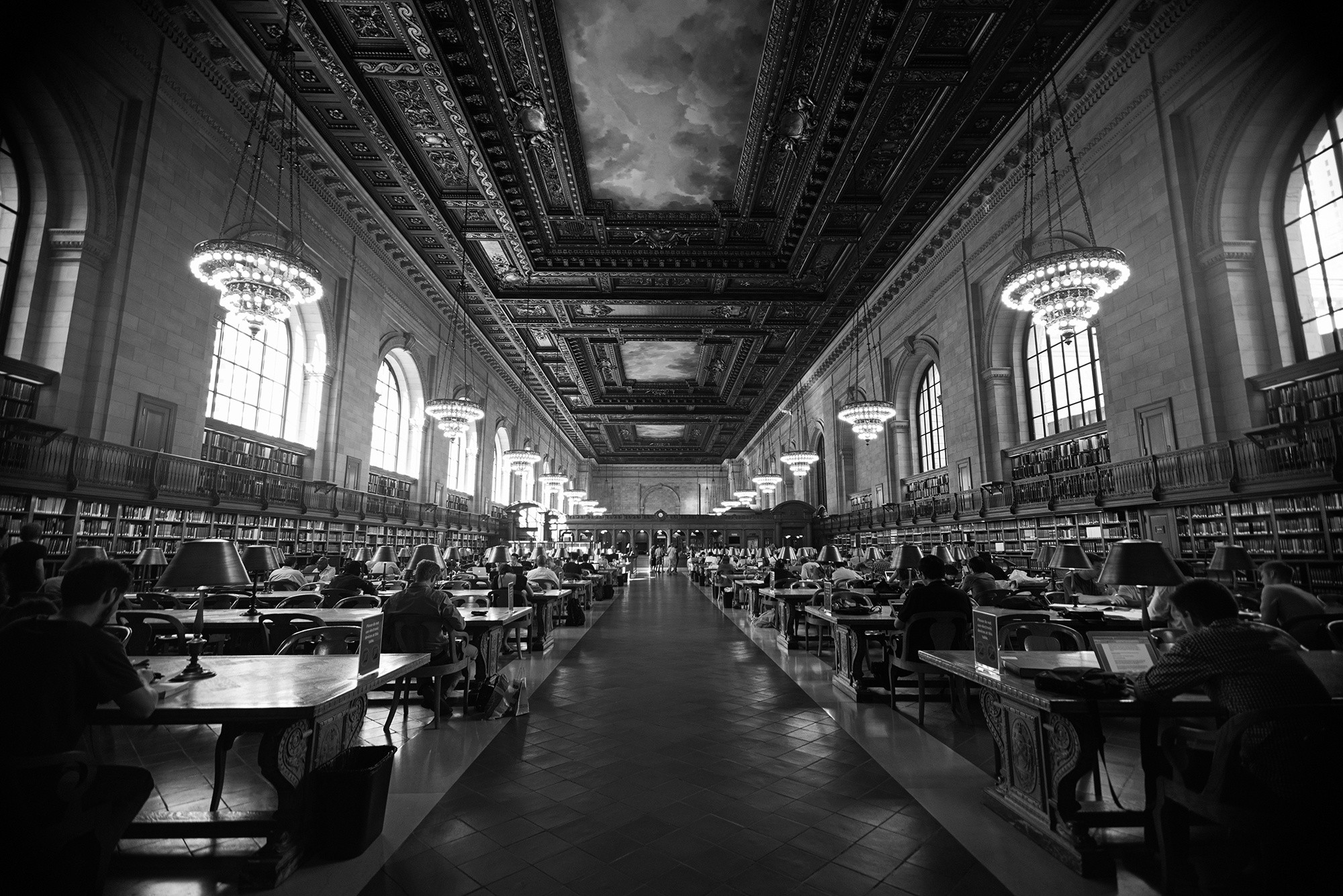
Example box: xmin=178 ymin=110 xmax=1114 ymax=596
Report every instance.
xmin=698 ymin=576 xmax=706 ymax=586
xmin=604 ymin=584 xmax=613 ymax=599
xmin=324 ymin=744 xmax=398 ymax=861
xmin=623 ymin=573 xmax=628 ymax=583
xmin=617 ymin=576 xmax=624 ymax=586
xmin=690 ymin=571 xmax=699 ymax=582
xmin=721 ymin=590 xmax=734 ymax=608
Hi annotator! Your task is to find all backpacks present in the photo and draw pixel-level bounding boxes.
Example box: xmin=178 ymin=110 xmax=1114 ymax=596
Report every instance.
xmin=565 ymin=597 xmax=586 ymax=626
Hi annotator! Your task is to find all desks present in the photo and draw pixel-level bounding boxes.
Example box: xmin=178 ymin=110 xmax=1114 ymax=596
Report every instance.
xmin=94 ymin=652 xmax=431 ymax=890
xmin=688 ymin=563 xmax=905 ymax=660
xmin=178 ymin=589 xmax=574 ymax=651
xmin=916 ymin=651 xmax=1343 ymax=882
xmin=437 ymin=554 xmax=639 ymax=612
xmin=109 ymin=607 xmax=535 ymax=704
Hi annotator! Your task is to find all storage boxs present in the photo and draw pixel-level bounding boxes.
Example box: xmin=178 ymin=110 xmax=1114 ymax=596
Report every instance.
xmin=972 ymin=605 xmax=1050 ymax=674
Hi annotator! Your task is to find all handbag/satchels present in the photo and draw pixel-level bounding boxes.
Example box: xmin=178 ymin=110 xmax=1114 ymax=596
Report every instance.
xmin=753 ymin=608 xmax=775 ymax=628
xmin=593 ymin=584 xmax=604 ymax=601
xmin=832 ymin=598 xmax=881 ymax=615
xmin=473 ymin=667 xmax=530 ymax=720
xmin=1035 ymin=666 xmax=1131 ymax=700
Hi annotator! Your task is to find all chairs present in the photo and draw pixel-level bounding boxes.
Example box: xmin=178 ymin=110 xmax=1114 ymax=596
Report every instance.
xmin=744 ymin=565 xmax=1185 ymax=802
xmin=0 ymin=749 xmax=117 ymax=896
xmin=1279 ymin=613 xmax=1343 ymax=650
xmin=1153 ymin=697 xmax=1343 ymax=896
xmin=102 ymin=570 xmax=593 ymax=812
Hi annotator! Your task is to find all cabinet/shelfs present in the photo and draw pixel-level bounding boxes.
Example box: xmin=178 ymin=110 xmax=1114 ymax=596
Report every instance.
xmin=0 ymin=371 xmax=42 ymax=472
xmin=1262 ymin=368 xmax=1343 ymax=470
xmin=1003 ymin=419 xmax=1115 ymax=500
xmin=0 ymin=471 xmax=503 ymax=592
xmin=830 ymin=465 xmax=1343 ymax=598
xmin=198 ymin=427 xmax=306 ymax=506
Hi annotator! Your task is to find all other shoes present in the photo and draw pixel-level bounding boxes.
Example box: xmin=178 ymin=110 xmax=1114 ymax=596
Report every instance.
xmin=552 ymin=620 xmax=559 ymax=626
xmin=423 ymin=692 xmax=454 ymax=717
xmin=586 ymin=600 xmax=591 ymax=610
xmin=869 ymin=660 xmax=896 ymax=691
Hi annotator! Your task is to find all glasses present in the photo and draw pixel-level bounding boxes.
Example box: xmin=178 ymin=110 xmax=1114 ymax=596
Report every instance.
xmin=315 ymin=562 xmax=326 ymax=566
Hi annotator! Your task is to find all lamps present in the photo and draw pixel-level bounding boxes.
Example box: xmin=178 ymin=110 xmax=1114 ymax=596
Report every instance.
xmin=837 ymin=146 xmax=898 ymax=446
xmin=778 ymin=538 xmax=1260 ymax=640
xmin=423 ymin=143 xmax=609 ymax=518
xmin=706 ymin=364 xmax=782 ymax=516
xmin=998 ymin=0 xmax=1133 ymax=345
xmin=778 ymin=281 xmax=821 ymax=481
xmin=189 ymin=0 xmax=323 ymax=340
xmin=58 ymin=538 xmax=602 ymax=680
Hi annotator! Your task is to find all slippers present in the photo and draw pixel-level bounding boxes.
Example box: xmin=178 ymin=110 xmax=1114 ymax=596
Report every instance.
xmin=502 ymin=648 xmax=514 ymax=655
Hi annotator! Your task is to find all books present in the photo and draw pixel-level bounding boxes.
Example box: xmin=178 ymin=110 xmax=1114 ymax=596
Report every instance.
xmin=152 ymin=679 xmax=190 ymax=699
xmin=1092 ymin=603 xmax=1130 ymax=612
xmin=20 ymin=423 xmax=507 ymax=555
xmin=820 ymin=369 xmax=1341 ymax=556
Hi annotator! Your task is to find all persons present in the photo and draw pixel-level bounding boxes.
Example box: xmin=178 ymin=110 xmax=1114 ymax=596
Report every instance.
xmin=268 ymin=558 xmax=311 ymax=592
xmin=489 ymin=565 xmax=535 ymax=653
xmin=871 ymin=554 xmax=974 ymax=691
xmin=370 ymin=561 xmax=402 ymax=580
xmin=302 ymin=555 xmax=323 ymax=572
xmin=961 ymin=555 xmax=997 ymax=604
xmin=685 ymin=541 xmax=926 ymax=594
xmin=1 ymin=522 xmax=48 ymax=598
xmin=1256 ymin=560 xmax=1326 ymax=646
xmin=324 ymin=560 xmax=379 ymax=608
xmin=1063 ymin=550 xmax=1129 ymax=604
xmin=981 ymin=552 xmax=1008 ymax=581
xmin=380 ymin=560 xmax=480 ymax=718
xmin=1118 ymin=585 xmax=1154 ymax=607
xmin=1145 ymin=560 xmax=1199 ymax=631
xmin=0 ymin=559 xmax=159 ymax=896
xmin=646 ymin=542 xmax=680 ymax=577
xmin=33 ymin=573 xmax=64 ymax=611
xmin=942 ymin=563 xmax=958 ymax=587
xmin=399 ymin=552 xmax=628 ymax=595
xmin=310 ymin=557 xmax=337 ymax=582
xmin=1128 ymin=580 xmax=1342 ymax=844
